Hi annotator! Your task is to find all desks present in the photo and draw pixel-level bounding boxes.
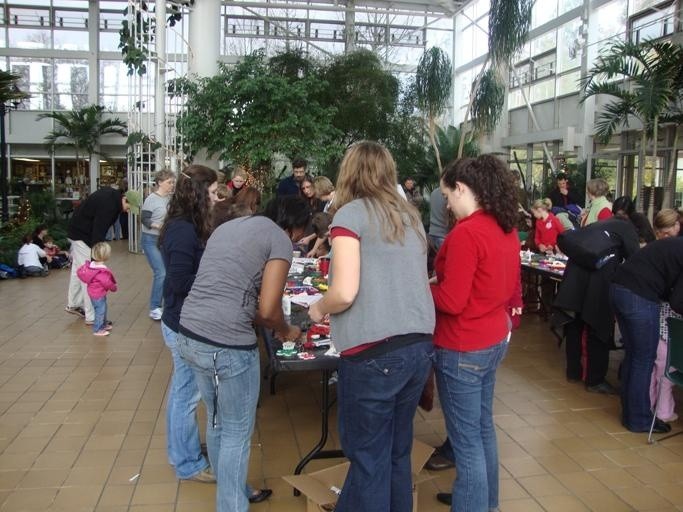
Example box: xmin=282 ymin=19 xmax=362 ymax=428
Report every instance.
xmin=519 ymin=250 xmax=568 ymax=322
xmin=259 ymin=240 xmax=345 ymax=497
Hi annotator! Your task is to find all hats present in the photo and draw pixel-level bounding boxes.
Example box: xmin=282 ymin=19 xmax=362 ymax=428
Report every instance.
xmin=125 ymin=190 xmax=140 ymax=214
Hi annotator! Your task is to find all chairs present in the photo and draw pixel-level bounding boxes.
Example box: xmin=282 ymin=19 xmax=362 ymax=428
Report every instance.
xmin=646 ymin=316 xmax=682 ymax=446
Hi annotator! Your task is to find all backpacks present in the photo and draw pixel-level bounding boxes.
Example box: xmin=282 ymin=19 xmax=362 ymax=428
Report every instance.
xmin=553 ymin=204 xmax=582 ymax=222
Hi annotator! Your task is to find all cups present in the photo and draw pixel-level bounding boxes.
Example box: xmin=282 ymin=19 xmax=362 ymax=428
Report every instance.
xmin=320 ymin=256 xmax=329 ymax=277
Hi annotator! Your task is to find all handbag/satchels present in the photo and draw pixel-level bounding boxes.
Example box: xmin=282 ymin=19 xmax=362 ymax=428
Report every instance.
xmin=556 ymin=229 xmax=615 ymax=270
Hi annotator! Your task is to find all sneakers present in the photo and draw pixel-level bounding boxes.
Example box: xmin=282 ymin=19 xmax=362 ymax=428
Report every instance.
xmin=86 ymin=321 xmax=112 ymax=336
xmin=567 ymin=365 xmax=583 ymax=382
xmin=622 ymin=416 xmax=671 ymax=432
xmin=64 ymin=305 xmax=84 ymax=316
xmin=150 ymin=308 xmax=162 ymax=320
xmin=187 ymin=468 xmax=216 ymax=483
xmin=588 ymin=382 xmax=620 ymax=394
xmin=200 ymin=443 xmax=207 ymax=454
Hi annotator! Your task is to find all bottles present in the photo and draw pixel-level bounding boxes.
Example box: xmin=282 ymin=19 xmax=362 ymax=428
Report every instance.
xmin=525 ymin=248 xmax=531 ymax=262
xmin=281 ymin=292 xmax=291 ymax=318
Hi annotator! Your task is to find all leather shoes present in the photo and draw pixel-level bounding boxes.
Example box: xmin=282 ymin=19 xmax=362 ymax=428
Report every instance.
xmin=437 ymin=494 xmax=452 ymax=505
xmin=423 ymin=447 xmax=455 ymax=469
xmin=249 ymin=489 xmax=272 ymax=502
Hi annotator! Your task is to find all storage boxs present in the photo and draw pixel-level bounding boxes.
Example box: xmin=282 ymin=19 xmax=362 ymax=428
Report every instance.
xmin=280 ymin=438 xmax=440 ymax=512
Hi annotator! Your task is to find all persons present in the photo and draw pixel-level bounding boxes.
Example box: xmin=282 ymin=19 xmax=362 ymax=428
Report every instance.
xmin=103 ymin=176 xmax=120 ymax=242
xmin=75 ymin=240 xmax=118 ymax=337
xmin=63 ymin=185 xmax=144 ymax=327
xmin=118 ymin=177 xmax=128 ymax=240
xmin=43 ymin=238 xmax=71 ymax=269
xmin=277 ymin=157 xmax=336 ymax=256
xmin=224 ymin=167 xmax=248 ymax=195
xmin=176 ymin=191 xmax=312 ymax=511
xmin=308 ymin=137 xmax=439 ymax=511
xmin=404 ymin=176 xmax=451 ymax=254
xmin=427 ymin=154 xmax=528 ymax=511
xmin=512 ymin=171 xmax=682 ymax=436
xmin=156 ymin=162 xmax=218 ymax=482
xmin=15 ymin=232 xmax=48 ymax=279
xmin=31 ymin=224 xmax=68 ymax=271
xmin=141 ymin=168 xmax=176 ymax=320
